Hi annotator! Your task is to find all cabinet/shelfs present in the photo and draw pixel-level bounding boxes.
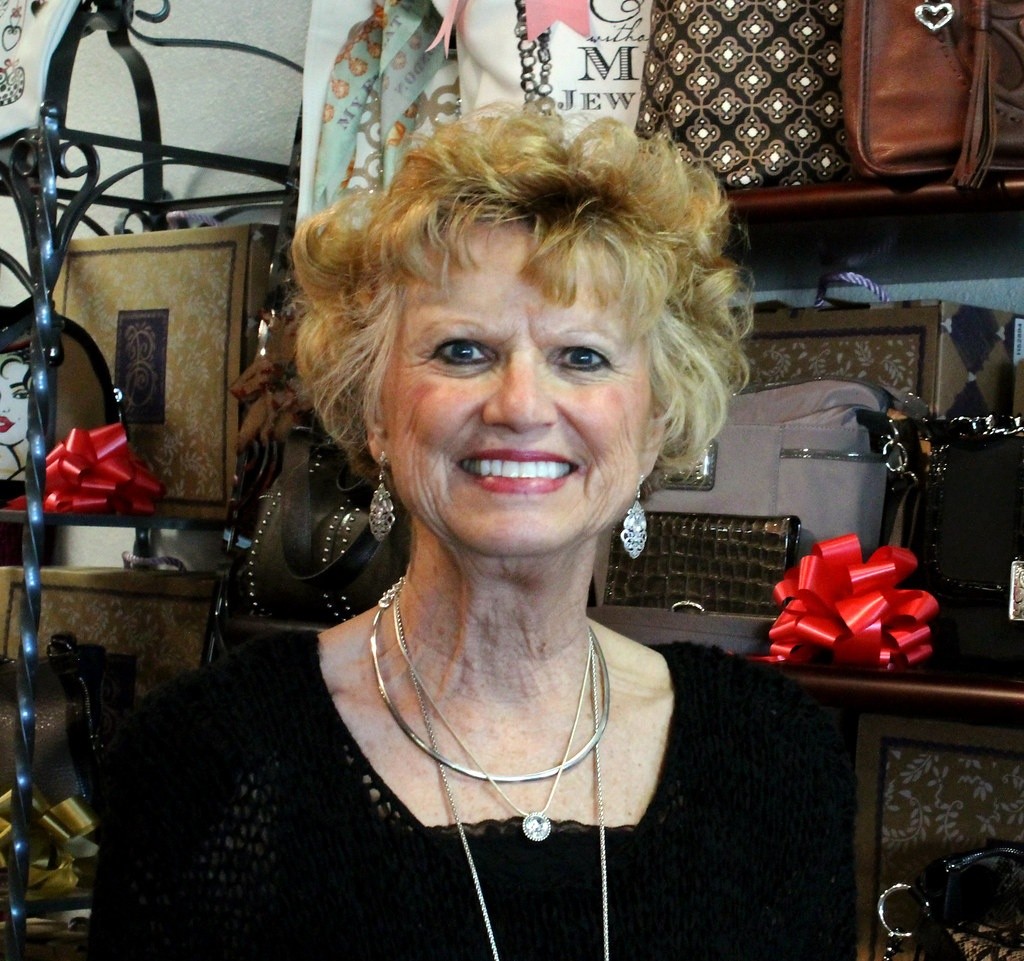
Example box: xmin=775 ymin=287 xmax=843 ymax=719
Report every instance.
xmin=221 ymin=176 xmax=1024 ymax=961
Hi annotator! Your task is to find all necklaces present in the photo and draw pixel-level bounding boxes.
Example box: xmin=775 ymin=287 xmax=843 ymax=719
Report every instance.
xmin=397 ymin=579 xmax=610 ymax=961
xmin=370 ymin=576 xmax=611 ymax=781
xmin=392 ymin=602 xmax=591 ymax=841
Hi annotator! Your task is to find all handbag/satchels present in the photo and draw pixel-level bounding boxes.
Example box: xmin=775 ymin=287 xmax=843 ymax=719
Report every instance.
xmin=419 ymin=0 xmax=1024 ymax=194
xmin=212 ymin=437 xmax=411 ymax=651
xmin=876 ymin=840 xmax=1024 ymax=961
xmin=603 ymin=376 xmax=929 ymax=614
xmin=1 ymin=632 xmax=133 ymax=842
xmin=872 ymin=410 xmax=1024 ymax=694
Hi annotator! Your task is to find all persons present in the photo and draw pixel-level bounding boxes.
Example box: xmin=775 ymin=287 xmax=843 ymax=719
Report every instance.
xmin=83 ymin=98 xmax=860 ymax=961
